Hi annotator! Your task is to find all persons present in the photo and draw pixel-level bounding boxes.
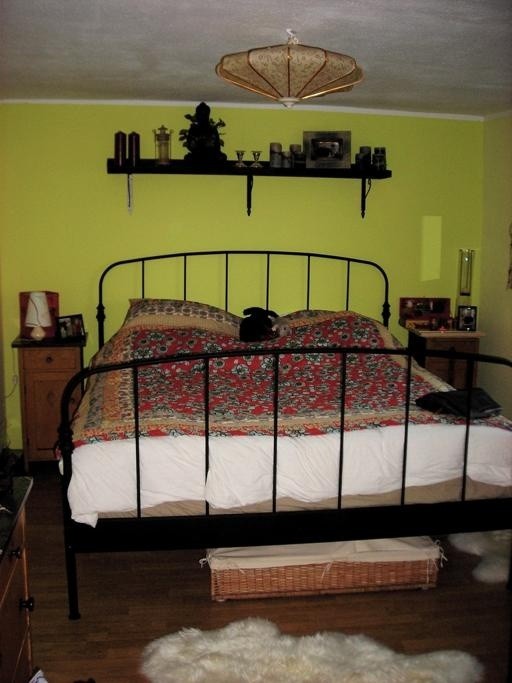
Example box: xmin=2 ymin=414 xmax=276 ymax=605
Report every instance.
xmin=464 ymin=309 xmax=473 ymax=324
xmin=60 ymin=318 xmax=82 ymax=337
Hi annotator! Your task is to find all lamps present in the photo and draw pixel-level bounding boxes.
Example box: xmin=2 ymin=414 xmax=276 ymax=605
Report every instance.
xmin=20 ymin=290 xmax=58 ymax=341
xmin=215 ymin=31 xmax=366 ymax=108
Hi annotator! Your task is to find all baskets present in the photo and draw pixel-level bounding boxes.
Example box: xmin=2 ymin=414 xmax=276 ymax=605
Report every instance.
xmin=209 ymin=558 xmax=439 ymax=602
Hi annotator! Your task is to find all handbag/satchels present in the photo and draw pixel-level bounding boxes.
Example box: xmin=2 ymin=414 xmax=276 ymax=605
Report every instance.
xmin=239 ymin=307 xmax=279 ymax=342
xmin=414 ymin=386 xmax=503 ymax=419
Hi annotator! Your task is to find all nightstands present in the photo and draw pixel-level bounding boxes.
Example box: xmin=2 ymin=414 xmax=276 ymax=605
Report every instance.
xmin=13 ymin=329 xmax=88 ymax=468
xmin=409 ymin=324 xmax=485 ymax=388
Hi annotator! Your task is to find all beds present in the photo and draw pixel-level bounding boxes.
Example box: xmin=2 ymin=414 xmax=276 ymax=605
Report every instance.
xmin=65 ymin=251 xmax=510 ymax=619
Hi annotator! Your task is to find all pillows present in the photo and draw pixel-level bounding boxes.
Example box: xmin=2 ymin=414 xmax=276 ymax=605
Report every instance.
xmin=124 ymin=296 xmax=242 ymax=331
xmin=277 ymin=310 xmax=340 ymax=326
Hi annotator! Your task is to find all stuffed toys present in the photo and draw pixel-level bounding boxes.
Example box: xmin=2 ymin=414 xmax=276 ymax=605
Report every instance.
xmin=239 ymin=307 xmax=292 ymax=343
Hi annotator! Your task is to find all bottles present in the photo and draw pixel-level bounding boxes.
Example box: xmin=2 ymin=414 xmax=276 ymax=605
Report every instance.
xmin=128 ymin=130 xmax=141 ymax=166
xmin=111 ymin=130 xmax=127 ymax=167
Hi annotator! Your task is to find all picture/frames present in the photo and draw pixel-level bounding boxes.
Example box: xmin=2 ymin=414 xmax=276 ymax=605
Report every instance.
xmin=58 ymin=313 xmax=86 ymax=339
xmin=457 ymin=305 xmax=477 ymax=331
xmin=302 ymin=130 xmax=351 ymax=168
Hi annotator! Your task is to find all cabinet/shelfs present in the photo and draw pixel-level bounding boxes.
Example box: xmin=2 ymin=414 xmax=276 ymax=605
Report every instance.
xmin=0 ymin=472 xmax=37 ymax=683
